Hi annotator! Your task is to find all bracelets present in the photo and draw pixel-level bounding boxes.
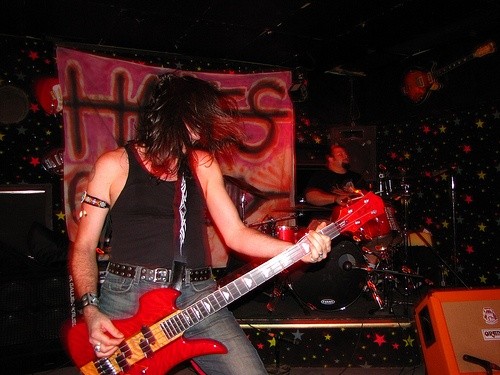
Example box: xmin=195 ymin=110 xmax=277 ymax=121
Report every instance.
xmin=74 ymin=291 xmax=100 ymax=320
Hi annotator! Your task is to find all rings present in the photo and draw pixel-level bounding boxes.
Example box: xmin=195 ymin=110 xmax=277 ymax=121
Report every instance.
xmin=310 ymin=255 xmax=318 ymax=263
xmin=318 ymin=253 xmax=323 ymax=258
xmin=94 ymin=342 xmax=101 ymax=352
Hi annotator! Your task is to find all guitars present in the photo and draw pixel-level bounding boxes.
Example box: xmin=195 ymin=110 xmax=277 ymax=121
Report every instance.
xmin=64 ymin=192 xmax=386 ymax=375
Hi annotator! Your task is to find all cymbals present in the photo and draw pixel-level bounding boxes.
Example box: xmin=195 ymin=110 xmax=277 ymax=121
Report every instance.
xmin=270 ymin=203 xmax=330 ymax=214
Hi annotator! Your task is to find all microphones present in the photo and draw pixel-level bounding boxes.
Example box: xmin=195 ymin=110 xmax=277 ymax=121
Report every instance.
xmin=343 ymin=261 xmax=352 ymax=272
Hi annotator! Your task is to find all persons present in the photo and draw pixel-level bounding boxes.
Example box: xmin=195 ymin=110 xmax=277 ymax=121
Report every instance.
xmin=366 ymin=67 xmax=446 ymax=124
xmin=304 ymin=142 xmax=378 ymax=265
xmin=68 ymin=71 xmax=332 ymax=375
xmin=0 ymin=76 xmax=64 ymax=184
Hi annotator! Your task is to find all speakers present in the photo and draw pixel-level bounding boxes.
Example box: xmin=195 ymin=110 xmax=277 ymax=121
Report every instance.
xmin=329 ymin=127 xmax=376 ymax=184
xmin=413 ymin=287 xmax=500 ymax=374
xmin=0 ymin=183 xmax=56 ymax=277
xmin=0 ymin=272 xmax=75 ymax=361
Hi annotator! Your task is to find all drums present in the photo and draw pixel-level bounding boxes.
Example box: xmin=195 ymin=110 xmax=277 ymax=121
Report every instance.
xmin=250 ymin=224 xmax=302 ymax=275
xmin=331 ymin=190 xmax=404 ymax=251
xmin=291 ymin=227 xmax=372 ymax=311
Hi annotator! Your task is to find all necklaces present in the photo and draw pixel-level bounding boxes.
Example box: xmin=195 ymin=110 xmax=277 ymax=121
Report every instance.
xmin=147 ymin=148 xmax=182 ymax=175
